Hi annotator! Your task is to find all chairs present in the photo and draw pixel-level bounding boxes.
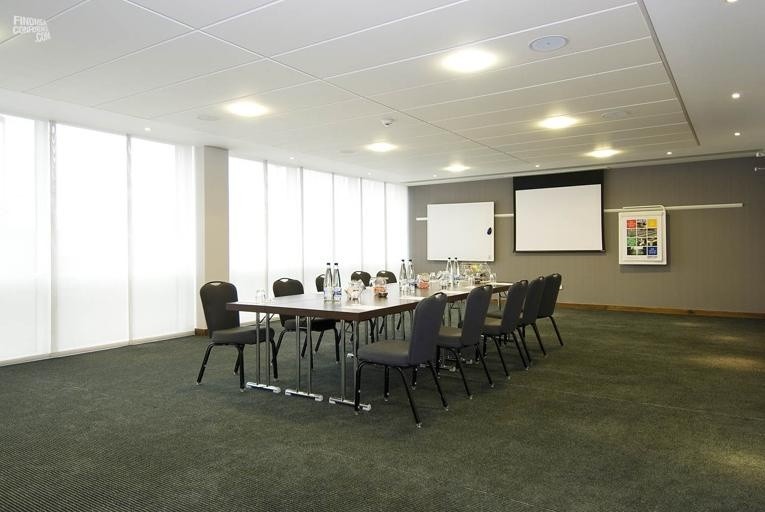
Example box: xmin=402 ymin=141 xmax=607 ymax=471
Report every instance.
xmin=352 ymin=292 xmax=449 ymax=425
xmin=351 ymin=272 xmax=385 ymax=335
xmin=483 ymin=275 xmax=550 ymax=363
xmin=457 ymin=279 xmax=529 ymax=381
xmin=504 ymin=272 xmax=564 ymax=355
xmin=197 ymin=281 xmax=279 ymax=392
xmin=270 ymin=277 xmax=341 ymax=366
xmin=377 ymin=271 xmax=405 ymax=331
xmin=316 ymin=273 xmax=376 ymax=345
xmin=412 ymin=284 xmax=495 ymax=400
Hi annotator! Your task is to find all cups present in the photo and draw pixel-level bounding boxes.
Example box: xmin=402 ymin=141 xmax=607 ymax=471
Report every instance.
xmin=430 ymin=272 xmax=436 ymax=280
xmin=399 ymin=281 xmax=410 ymax=295
xmin=255 ymin=290 xmax=267 ymax=303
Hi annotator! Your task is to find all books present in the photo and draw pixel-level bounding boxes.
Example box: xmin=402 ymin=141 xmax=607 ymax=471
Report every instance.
xmin=487 ymin=282 xmax=513 ymax=286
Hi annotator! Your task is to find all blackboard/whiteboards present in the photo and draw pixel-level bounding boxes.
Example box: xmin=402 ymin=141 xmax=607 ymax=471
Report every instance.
xmin=427 ymin=202 xmax=494 ymax=262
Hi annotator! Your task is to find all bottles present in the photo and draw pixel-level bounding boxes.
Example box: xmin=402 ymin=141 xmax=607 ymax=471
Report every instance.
xmin=323 ymin=263 xmax=333 ymax=301
xmin=332 ymin=263 xmax=342 ymax=301
xmin=437 ymin=255 xmax=496 ymax=290
xmin=399 ymin=259 xmax=408 ymax=290
xmin=407 ymin=259 xmax=416 ymax=291
